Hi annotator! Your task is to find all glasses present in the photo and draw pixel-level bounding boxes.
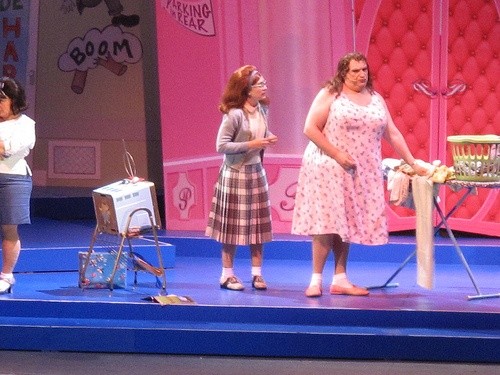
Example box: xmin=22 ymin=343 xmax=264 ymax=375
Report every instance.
xmin=250 ymin=80 xmax=269 ymax=89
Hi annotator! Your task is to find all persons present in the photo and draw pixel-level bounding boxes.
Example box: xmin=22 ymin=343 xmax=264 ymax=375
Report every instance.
xmin=0 ymin=76 xmax=36 ymax=295
xmin=304 ymin=51 xmax=426 ymax=298
xmin=215 ymin=64 xmax=278 ymax=291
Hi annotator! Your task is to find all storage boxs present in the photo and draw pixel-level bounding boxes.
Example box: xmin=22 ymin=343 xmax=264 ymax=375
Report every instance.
xmin=79 ymin=252 xmax=127 ymax=289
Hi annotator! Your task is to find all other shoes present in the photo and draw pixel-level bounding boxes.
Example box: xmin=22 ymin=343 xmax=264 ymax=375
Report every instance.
xmin=305 ymin=282 xmax=324 ymax=297
xmin=251 ymin=275 xmax=268 ymax=289
xmin=329 ymin=276 xmax=370 ymax=296
xmin=219 ymin=277 xmax=246 ymax=291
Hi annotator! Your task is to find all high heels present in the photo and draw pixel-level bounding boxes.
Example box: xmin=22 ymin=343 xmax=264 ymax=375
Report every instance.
xmin=0 ymin=272 xmax=15 ymax=294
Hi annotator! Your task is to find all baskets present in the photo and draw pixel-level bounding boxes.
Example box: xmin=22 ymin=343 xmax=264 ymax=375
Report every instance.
xmin=447 ymin=134 xmax=500 ymax=182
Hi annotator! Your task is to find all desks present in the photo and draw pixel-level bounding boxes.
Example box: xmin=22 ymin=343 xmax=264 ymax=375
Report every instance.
xmin=365 ymin=168 xmax=500 ymax=301
